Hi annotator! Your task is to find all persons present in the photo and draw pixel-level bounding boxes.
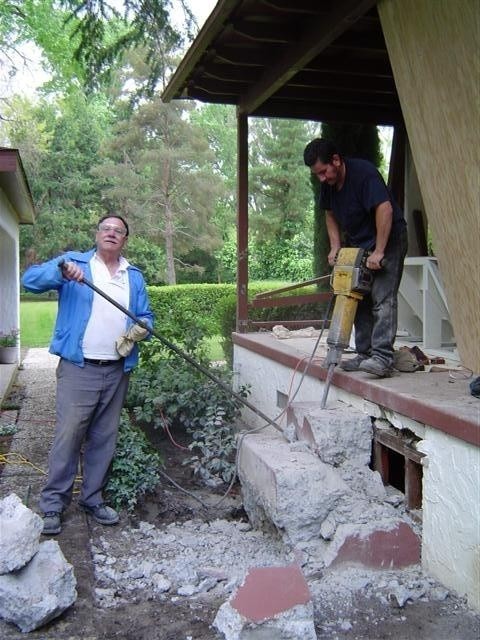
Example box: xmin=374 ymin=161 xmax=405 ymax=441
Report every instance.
xmin=20 ymin=213 xmax=154 ymax=537
xmin=302 ymin=137 xmax=404 ymax=377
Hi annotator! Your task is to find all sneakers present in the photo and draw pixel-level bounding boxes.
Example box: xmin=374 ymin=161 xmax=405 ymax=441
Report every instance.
xmin=86 ymin=502 xmax=121 ymax=526
xmin=40 ymin=510 xmax=62 ymax=536
xmin=339 ymin=352 xmax=370 ymax=371
xmin=359 ymin=354 xmax=392 ymax=378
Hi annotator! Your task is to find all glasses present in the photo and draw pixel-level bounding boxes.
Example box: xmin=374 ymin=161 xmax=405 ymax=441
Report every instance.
xmin=98 ymin=222 xmax=127 ymax=238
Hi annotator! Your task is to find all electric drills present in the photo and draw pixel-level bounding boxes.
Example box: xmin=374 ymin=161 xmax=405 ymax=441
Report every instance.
xmin=319 ymin=247 xmax=388 ymax=410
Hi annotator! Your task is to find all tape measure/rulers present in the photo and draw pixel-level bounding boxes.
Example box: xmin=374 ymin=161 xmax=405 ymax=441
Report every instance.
xmin=431 ymin=357 xmax=445 ymax=364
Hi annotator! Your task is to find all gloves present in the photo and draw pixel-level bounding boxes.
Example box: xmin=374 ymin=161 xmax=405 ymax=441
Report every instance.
xmin=116 ymin=320 xmax=149 ymax=358
